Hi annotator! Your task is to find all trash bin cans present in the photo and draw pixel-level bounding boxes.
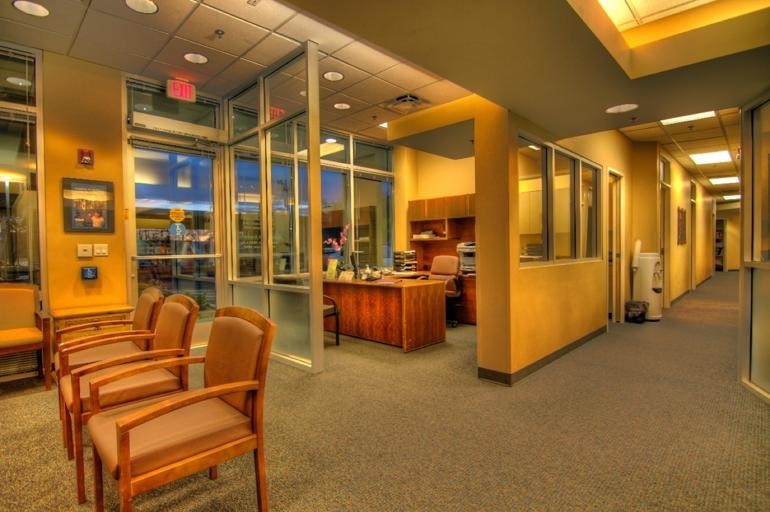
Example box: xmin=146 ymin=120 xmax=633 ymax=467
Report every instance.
xmin=624 ymin=301 xmax=649 ymax=323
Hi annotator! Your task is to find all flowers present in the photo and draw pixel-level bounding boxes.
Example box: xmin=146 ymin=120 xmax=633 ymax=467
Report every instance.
xmin=323 ymin=224 xmax=350 ymax=254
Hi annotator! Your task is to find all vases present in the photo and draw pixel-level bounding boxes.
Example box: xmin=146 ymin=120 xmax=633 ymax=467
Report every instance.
xmin=326 ymin=254 xmax=344 ymax=282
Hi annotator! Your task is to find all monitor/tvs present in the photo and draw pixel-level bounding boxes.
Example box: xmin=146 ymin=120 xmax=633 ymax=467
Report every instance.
xmin=350 ymin=252 xmax=358 ymax=279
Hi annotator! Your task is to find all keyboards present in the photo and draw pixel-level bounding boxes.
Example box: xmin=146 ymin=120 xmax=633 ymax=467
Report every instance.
xmin=366 ymin=276 xmax=377 ymax=281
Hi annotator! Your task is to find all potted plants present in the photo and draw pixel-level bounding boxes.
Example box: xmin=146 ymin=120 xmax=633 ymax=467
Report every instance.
xmin=192 ymin=292 xmax=216 ymax=323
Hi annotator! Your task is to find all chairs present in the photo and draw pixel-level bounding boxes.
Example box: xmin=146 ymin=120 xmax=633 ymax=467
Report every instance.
xmin=58 ymin=293 xmax=201 ymax=506
xmin=71 ymin=304 xmax=276 ymax=512
xmin=54 ymin=287 xmax=167 ymax=449
xmin=323 ymin=295 xmax=339 ymax=345
xmin=414 ymin=255 xmax=462 ymax=328
xmin=0 ymin=283 xmax=52 ymax=393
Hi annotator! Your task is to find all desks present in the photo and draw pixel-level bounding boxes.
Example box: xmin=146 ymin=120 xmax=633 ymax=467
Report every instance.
xmin=322 ymin=275 xmax=446 ymax=353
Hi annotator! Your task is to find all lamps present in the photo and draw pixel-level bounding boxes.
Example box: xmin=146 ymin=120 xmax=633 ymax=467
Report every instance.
xmin=736 ymin=147 xmax=741 ymax=160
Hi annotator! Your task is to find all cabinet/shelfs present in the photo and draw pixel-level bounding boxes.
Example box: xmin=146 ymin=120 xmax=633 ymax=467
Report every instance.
xmin=137 ymin=207 xmax=307 ymax=291
xmin=323 ymin=206 xmax=384 ymax=270
xmin=447 ymin=276 xmax=476 ymax=325
xmin=407 ymin=193 xmax=476 ymax=275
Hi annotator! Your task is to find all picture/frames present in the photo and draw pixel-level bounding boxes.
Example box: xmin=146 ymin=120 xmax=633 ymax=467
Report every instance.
xmin=716 ymin=218 xmax=728 ymax=273
xmin=62 ymin=177 xmax=115 ymax=233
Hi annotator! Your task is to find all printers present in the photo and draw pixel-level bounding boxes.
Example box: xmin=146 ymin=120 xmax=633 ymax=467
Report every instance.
xmin=456 ymin=242 xmax=476 ymax=271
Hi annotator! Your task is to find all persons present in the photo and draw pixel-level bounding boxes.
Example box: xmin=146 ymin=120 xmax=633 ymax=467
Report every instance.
xmin=92 ymin=208 xmax=104 ymax=227
